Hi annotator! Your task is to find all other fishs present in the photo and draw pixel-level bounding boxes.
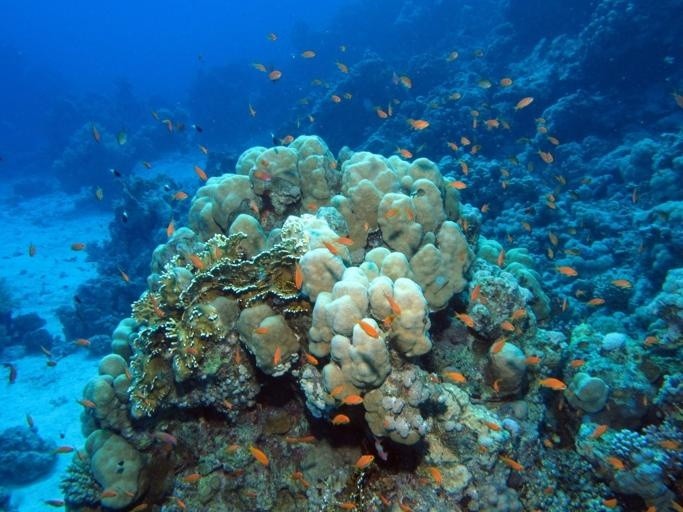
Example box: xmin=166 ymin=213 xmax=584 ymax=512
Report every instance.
xmin=0 ymin=33 xmax=683 ymax=512
